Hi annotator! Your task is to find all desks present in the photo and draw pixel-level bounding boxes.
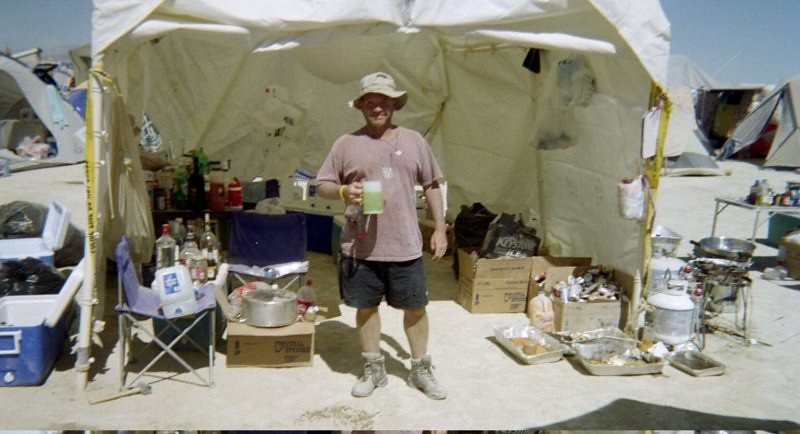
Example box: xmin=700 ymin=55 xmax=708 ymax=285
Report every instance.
xmin=711 ymin=197 xmax=800 ymax=246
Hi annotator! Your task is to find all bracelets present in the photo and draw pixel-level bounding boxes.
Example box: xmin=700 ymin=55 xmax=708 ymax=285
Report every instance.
xmin=339 ymin=185 xmax=346 ymax=201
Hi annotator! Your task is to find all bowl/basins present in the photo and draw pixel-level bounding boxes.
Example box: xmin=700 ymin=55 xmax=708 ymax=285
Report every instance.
xmin=241 ymin=288 xmax=297 ymax=327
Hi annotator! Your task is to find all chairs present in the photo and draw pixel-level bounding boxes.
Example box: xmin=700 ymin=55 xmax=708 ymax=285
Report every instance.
xmin=115 ymin=236 xmax=216 ymax=389
xmin=224 ymin=211 xmax=309 ymax=292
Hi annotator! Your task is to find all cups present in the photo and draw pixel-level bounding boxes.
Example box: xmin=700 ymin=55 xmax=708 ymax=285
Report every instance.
xmin=361 ymin=181 xmax=383 ymax=215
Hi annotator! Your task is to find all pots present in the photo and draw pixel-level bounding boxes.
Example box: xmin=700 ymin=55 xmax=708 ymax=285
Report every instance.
xmin=690 ymin=237 xmax=756 ymax=262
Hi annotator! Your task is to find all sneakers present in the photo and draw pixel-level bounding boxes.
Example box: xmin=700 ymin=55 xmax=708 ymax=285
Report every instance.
xmin=407 ymin=358 xmax=445 ymax=399
xmin=352 ymin=356 xmax=389 ymax=397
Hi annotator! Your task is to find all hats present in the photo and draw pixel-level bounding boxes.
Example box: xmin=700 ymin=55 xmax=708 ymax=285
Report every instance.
xmin=348 ymin=71 xmax=407 ymax=111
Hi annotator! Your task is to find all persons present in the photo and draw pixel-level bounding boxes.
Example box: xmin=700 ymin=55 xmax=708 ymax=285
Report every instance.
xmin=316 ymin=72 xmax=448 ymax=400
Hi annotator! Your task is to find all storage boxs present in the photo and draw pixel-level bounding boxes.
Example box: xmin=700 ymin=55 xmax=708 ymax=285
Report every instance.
xmin=217 ymin=288 xmax=342 ymax=368
xmin=1 ymin=201 xmax=70 ymax=268
xmin=420 ymin=220 xmax=453 ymax=255
xmin=457 ymin=245 xmax=623 ymax=331
xmin=332 ymin=215 xmax=348 ymax=256
xmin=0 ymin=256 xmax=84 ymax=387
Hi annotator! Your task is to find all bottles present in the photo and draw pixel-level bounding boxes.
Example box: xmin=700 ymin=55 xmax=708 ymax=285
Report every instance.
xmin=297 ymin=280 xmax=315 ymax=315
xmin=749 ymin=179 xmax=773 ymax=205
xmin=144 ymin=147 xmax=243 ymax=213
xmin=156 ymin=213 xmax=220 ymax=284
xmin=527 ymin=290 xmax=554 ymax=333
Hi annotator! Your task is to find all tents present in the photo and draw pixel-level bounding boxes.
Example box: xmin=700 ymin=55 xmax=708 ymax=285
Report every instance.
xmin=666 ymin=54 xmax=715 ymax=114
xmin=75 ymin=0 xmax=672 ymax=389
xmin=717 ymin=78 xmax=800 ymax=168
xmin=0 ymin=51 xmax=90 ymax=173
xmin=658 ymin=87 xmax=724 ymax=176
xmin=703 ymin=84 xmax=767 ymax=142
